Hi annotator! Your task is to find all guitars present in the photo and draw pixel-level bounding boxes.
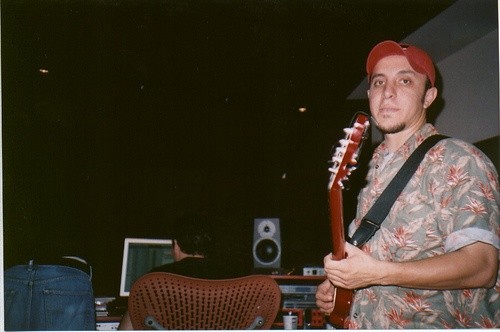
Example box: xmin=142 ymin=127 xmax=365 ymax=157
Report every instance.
xmin=328 ymin=114 xmax=370 ymax=330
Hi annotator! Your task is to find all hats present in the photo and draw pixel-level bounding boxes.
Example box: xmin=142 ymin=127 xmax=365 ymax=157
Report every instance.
xmin=366 ymin=41 xmax=436 ymax=87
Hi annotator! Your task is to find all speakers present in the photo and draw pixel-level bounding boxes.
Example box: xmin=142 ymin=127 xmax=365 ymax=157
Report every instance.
xmin=252 ymin=218 xmax=281 ymax=274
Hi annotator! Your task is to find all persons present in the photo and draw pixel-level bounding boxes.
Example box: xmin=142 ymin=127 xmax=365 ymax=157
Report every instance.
xmin=314 ymin=39 xmax=500 ymax=330
xmin=5 ymin=248 xmax=97 ymax=330
xmin=115 ymin=217 xmax=243 ymax=331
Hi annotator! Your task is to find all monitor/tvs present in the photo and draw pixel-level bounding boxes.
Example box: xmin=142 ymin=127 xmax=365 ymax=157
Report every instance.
xmin=119 ymin=238 xmax=175 ymax=297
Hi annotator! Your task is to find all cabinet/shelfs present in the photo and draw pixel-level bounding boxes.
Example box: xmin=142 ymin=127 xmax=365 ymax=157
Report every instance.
xmin=267 ymin=275 xmax=329 ymax=330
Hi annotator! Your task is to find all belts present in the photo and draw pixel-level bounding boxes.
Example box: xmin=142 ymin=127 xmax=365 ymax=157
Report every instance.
xmin=38 ymin=256 xmax=90 ymax=274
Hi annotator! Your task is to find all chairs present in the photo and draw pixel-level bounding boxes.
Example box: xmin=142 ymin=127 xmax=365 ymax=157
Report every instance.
xmin=129 ymin=271 xmax=282 ymax=330
